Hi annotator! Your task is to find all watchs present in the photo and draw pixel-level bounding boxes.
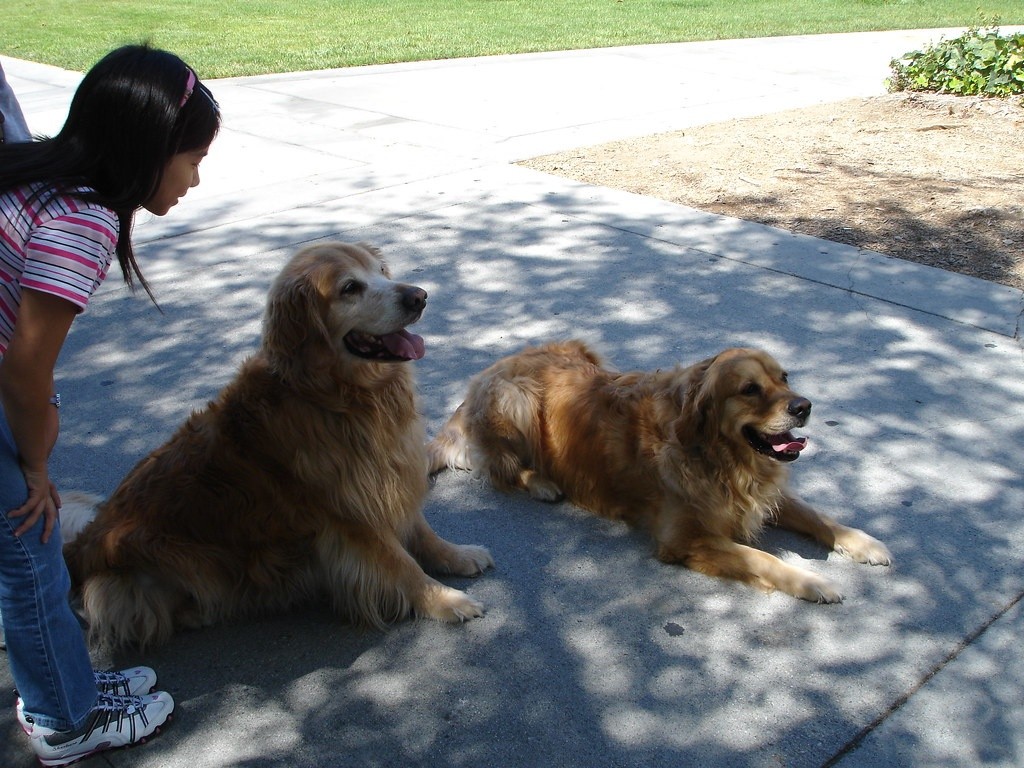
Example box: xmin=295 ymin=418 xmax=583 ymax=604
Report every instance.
xmin=50 ymin=393 xmax=61 ymax=407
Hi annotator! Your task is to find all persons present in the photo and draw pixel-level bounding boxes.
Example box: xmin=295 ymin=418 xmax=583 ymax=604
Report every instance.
xmin=0 ymin=43 xmax=220 ymax=768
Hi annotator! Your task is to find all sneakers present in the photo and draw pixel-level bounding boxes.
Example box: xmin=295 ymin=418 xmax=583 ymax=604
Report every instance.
xmin=14 ymin=666 xmax=158 ymax=737
xmin=25 ymin=691 xmax=175 ymax=767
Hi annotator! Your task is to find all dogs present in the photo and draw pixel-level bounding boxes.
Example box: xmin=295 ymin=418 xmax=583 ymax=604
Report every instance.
xmin=61 ymin=240 xmax=494 ymax=663
xmin=424 ymin=340 xmax=894 ymax=603
